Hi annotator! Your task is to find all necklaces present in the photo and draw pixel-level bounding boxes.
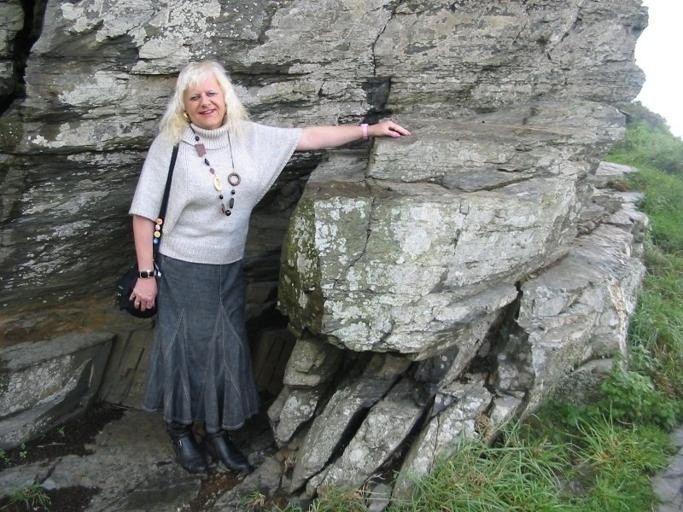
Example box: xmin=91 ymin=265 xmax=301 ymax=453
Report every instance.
xmin=191 ymin=128 xmax=240 ymax=218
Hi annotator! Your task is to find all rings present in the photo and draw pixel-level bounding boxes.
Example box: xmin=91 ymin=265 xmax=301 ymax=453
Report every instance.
xmin=134 ymin=298 xmax=140 ymax=305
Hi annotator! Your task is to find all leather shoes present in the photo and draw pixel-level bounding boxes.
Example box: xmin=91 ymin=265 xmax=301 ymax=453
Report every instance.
xmin=175 ymin=431 xmax=249 ymax=473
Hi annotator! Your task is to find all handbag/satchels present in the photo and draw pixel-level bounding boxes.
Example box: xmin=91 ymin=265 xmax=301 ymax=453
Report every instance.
xmin=119 ymin=264 xmax=158 ymax=318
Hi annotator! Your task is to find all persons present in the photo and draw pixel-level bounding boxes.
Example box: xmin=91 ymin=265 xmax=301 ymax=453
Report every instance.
xmin=125 ymin=57 xmax=413 ymax=476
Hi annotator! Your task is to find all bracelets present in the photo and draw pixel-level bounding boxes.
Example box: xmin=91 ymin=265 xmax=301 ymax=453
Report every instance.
xmin=135 ymin=270 xmax=156 ymax=279
xmin=358 ymin=122 xmax=369 ymax=140
